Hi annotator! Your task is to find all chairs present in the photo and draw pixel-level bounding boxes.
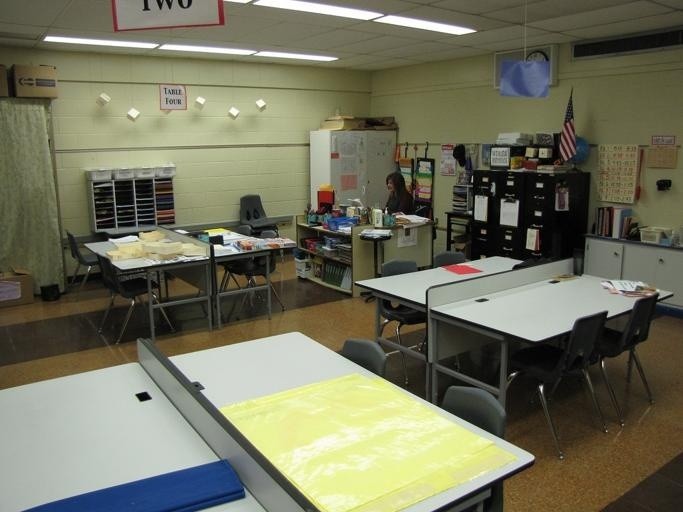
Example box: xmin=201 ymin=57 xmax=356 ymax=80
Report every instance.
xmin=217 ymin=192 xmax=289 ymax=325
xmin=602 ymin=290 xmax=661 ymax=430
xmin=65 ymin=230 xmax=110 ymax=291
xmin=98 ymin=255 xmax=181 ymax=346
xmin=440 ymin=381 xmax=507 ymax=447
xmin=529 ymin=307 xmax=610 ymax=435
xmin=340 ymin=336 xmax=388 ymax=382
xmin=377 ymin=257 xmax=428 ymax=346
xmin=432 ymin=253 xmax=465 ymax=267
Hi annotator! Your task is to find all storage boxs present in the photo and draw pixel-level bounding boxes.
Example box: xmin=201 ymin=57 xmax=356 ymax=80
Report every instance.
xmin=0 ymin=64 xmax=12 ymax=95
xmin=640 ymin=226 xmax=672 ymax=245
xmin=0 ymin=266 xmax=36 ymax=309
xmin=11 ymin=61 xmax=60 ymax=101
xmin=84 ymin=167 xmax=112 ymax=183
xmin=489 ymin=143 xmax=530 ymax=169
xmin=112 ymin=166 xmax=134 ymax=179
xmin=134 ymin=166 xmax=157 ymax=178
xmin=153 ymin=163 xmax=178 ymax=178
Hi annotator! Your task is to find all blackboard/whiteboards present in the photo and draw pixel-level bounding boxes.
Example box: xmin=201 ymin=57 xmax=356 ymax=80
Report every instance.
xmin=396 ymin=143 xmax=683 ymax=251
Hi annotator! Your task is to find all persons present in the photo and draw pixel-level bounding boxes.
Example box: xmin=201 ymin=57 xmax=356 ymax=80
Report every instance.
xmin=366 ymin=173 xmax=412 ymax=216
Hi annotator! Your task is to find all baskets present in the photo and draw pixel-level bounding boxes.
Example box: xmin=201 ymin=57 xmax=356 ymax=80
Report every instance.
xmin=638 ymin=225 xmax=673 ymax=245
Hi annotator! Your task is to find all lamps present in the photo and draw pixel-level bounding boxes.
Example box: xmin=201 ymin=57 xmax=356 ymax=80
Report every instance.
xmin=526 ymin=49 xmax=549 ymax=62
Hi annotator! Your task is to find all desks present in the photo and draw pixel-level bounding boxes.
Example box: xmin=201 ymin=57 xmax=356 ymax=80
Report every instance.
xmin=83 ymin=235 xmax=212 ymax=339
xmin=430 ymin=269 xmax=672 ymax=437
xmin=1 ymin=362 xmax=271 ymax=511
xmin=375 ymin=220 xmax=438 ymax=277
xmin=355 ymin=253 xmax=540 ymax=400
xmin=442 ymin=209 xmax=472 ymax=261
xmin=200 ymin=228 xmax=298 ymax=322
xmin=168 ymin=330 xmax=536 ymax=511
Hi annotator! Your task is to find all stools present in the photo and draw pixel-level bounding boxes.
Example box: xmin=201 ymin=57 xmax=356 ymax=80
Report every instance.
xmin=358 ymin=231 xmax=393 ymax=303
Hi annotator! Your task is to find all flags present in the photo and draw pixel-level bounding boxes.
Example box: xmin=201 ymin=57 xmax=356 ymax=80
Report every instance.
xmin=559 ymin=92 xmax=577 ymax=161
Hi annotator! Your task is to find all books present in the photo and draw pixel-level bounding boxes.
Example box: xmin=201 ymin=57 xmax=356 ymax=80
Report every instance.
xmin=595 ymin=205 xmax=634 ymax=240
xmin=537 ymin=165 xmax=569 ymax=174
xmin=450 ymin=185 xmax=469 ymax=253
xmin=602 ymin=278 xmax=658 ymax=298
xmin=104 ymin=227 xmax=298 ymax=262
xmin=92 ymin=177 xmax=175 ymax=230
xmin=347 ymin=198 xmax=363 ymax=207
xmin=323 ymin=243 xmax=351 ymax=288
xmin=525 ymin=228 xmax=540 ymax=251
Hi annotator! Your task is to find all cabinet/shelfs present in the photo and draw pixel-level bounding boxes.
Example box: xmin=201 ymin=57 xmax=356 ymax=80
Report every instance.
xmin=623 ymin=241 xmax=683 ymax=313
xmin=293 ymin=216 xmax=375 ymax=298
xmin=308 ymin=129 xmax=398 ymax=210
xmin=584 ymin=236 xmax=623 ymax=288
xmin=471 ymin=171 xmax=591 ymax=267
xmin=86 ymin=177 xmax=176 ymax=234
